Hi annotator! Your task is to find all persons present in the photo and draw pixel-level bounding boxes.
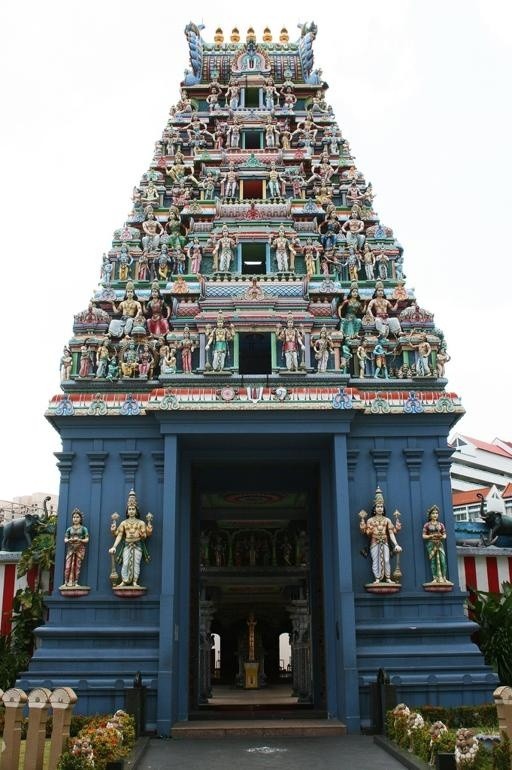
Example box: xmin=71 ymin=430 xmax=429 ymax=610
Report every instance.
xmin=365 ymin=501 xmax=403 ymax=583
xmin=421 ymin=504 xmax=447 ymax=582
xmin=63 ymin=506 xmax=91 ymax=586
xmin=108 ymin=504 xmax=147 ymax=587
